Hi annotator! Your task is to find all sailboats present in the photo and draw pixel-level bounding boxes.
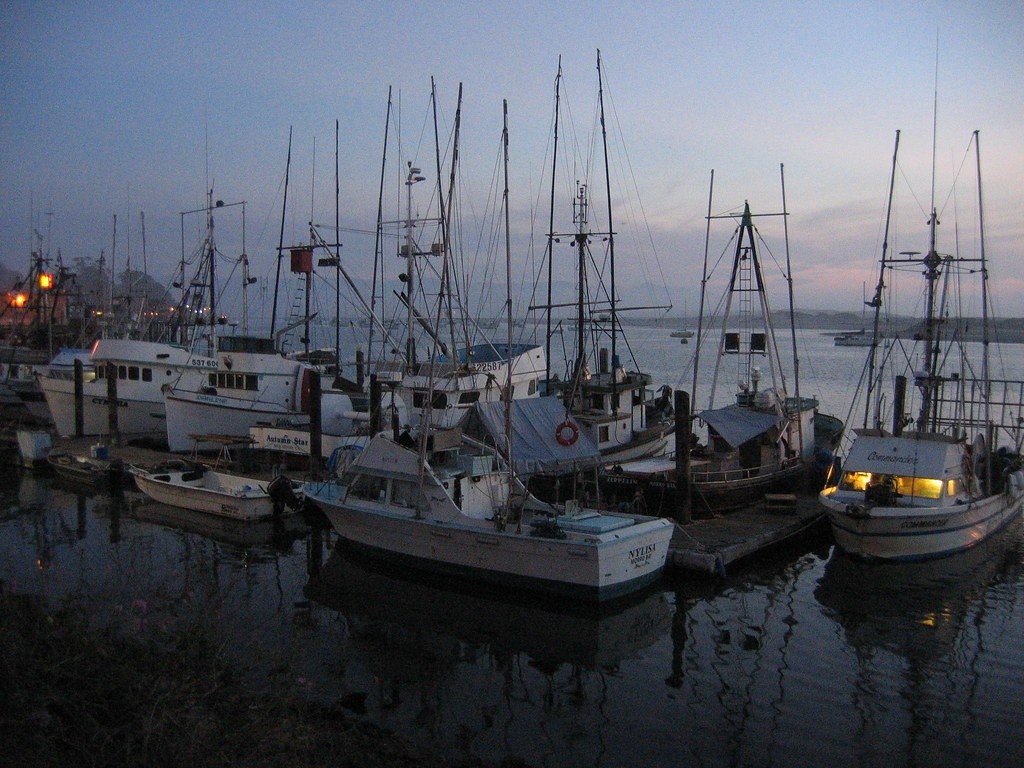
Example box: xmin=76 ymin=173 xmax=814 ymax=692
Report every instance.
xmin=298 ymin=80 xmax=678 ymax=605
xmin=670 ymin=298 xmax=694 ymax=338
xmin=666 ymin=163 xmax=846 ymax=575
xmin=0 ymin=48 xmax=674 ymax=516
xmin=834 ymin=281 xmax=883 ymax=346
xmin=820 ymin=25 xmax=1023 ymax=566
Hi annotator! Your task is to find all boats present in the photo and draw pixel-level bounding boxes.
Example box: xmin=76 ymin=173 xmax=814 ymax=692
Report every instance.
xmin=128 ymin=458 xmax=310 ymax=523
xmin=46 ymin=442 xmax=123 ymax=489
xmin=681 ymin=338 xmax=688 ymax=344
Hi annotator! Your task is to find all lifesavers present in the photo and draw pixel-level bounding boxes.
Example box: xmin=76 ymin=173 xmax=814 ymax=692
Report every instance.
xmin=555 ymin=421 xmax=580 ymax=445
